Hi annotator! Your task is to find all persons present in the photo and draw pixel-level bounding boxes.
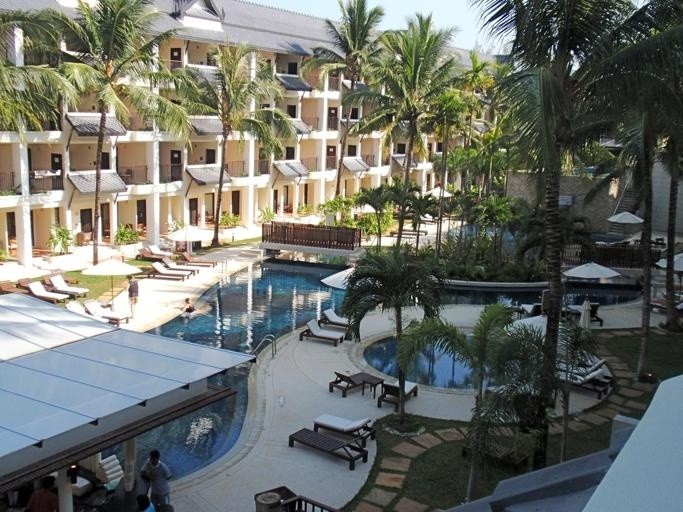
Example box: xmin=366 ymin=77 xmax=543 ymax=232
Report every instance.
xmin=182 ymin=298 xmax=209 ymax=316
xmin=3 ymin=449 xmax=174 ymax=512
xmin=126 ymin=276 xmax=139 ymax=319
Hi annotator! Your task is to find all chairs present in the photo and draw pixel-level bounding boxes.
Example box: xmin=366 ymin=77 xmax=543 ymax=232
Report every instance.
xmin=317 ymin=308 xmax=354 ymax=329
xmin=312 ymin=413 xmax=376 ymax=448
xmin=610 ymin=234 xmax=666 ymax=249
xmin=376 ymin=380 xmax=417 ymax=413
xmin=328 ymin=371 xmax=373 ymax=398
xmin=509 ymin=286 xmax=682 ymax=327
xmin=288 ymin=427 xmax=368 ymax=471
xmin=298 ymin=318 xmax=344 ymax=346
xmin=0 ymin=243 xmax=217 ymax=327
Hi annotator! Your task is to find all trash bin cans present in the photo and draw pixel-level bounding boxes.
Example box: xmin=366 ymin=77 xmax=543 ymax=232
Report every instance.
xmin=254 ymin=486 xmax=297 ymax=512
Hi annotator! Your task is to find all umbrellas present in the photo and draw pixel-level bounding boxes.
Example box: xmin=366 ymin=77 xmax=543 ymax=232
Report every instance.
xmin=354 ymin=204 xmax=387 ymax=214
xmin=423 ymin=186 xmax=452 ymax=200
xmin=576 ymin=297 xmax=591 ymax=340
xmin=167 ymin=225 xmax=214 ymax=259
xmin=320 ymin=267 xmax=383 ymax=290
xmin=654 ymin=253 xmax=683 ymax=295
xmin=81 ymin=258 xmax=143 ymax=312
xmin=561 ymin=261 xmax=622 ymax=298
xmin=607 ymin=211 xmax=644 ymax=240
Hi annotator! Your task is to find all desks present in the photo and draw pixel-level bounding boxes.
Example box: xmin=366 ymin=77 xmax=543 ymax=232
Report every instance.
xmin=361 ymin=376 xmax=384 ymax=399
